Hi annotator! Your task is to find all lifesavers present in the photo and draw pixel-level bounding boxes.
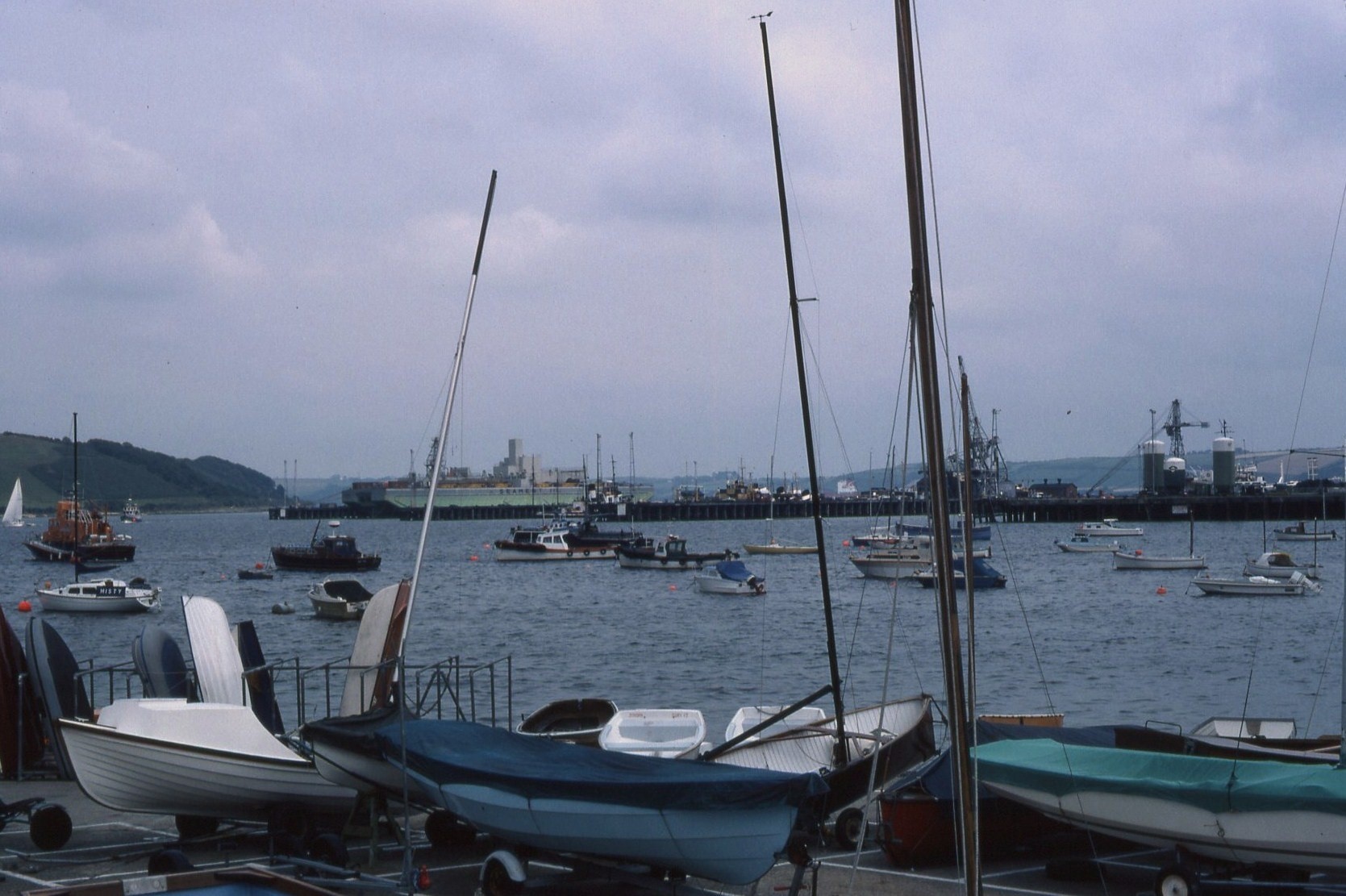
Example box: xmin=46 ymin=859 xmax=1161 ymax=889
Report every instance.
xmin=661 ymin=558 xmax=667 ymax=564
xmin=678 ymin=559 xmax=686 ymax=565
xmin=583 ymin=549 xmax=590 ymax=556
xmin=695 ymin=558 xmax=703 ymax=566
xmin=600 ymin=548 xmax=606 ymax=554
xmin=567 ymin=550 xmax=573 ymax=556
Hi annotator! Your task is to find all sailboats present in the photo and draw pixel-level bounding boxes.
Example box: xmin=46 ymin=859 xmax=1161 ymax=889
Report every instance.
xmin=850 ymin=356 xmax=1030 ymax=591
xmin=1 ymin=475 xmax=26 ymax=528
xmin=21 ymin=412 xmax=165 ymax=613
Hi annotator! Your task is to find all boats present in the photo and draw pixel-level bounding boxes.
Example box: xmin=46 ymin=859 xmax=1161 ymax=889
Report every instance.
xmin=1190 ymin=485 xmax=1336 ymax=596
xmin=236 ymin=521 xmax=383 ymax=621
xmin=56 ymin=699 xmax=386 ymax=822
xmin=340 ymin=576 xmax=415 ymax=717
xmin=490 ymin=430 xmax=766 ymax=596
xmin=132 ymin=595 xmax=288 ymax=738
xmin=1111 ymin=551 xmax=1208 ymax=570
xmin=119 ymin=498 xmax=143 ymax=522
xmin=1185 ymin=419 xmax=1302 ymax=496
xmin=1052 ymin=518 xmax=1146 ymax=553
xmin=742 ymin=543 xmax=819 ymax=554
xmin=303 ymin=696 xmax=954 ymax=885
xmin=971 ymin=736 xmax=1346 ymax=881
xmin=25 ymin=616 xmax=94 ymax=781
